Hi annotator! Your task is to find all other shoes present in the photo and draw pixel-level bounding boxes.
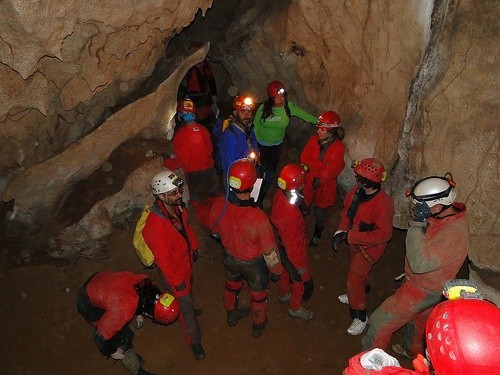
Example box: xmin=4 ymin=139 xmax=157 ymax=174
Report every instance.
xmin=191 ymin=333 xmax=207 ymax=360
xmin=227 ymin=304 xmax=250 ymax=327
xmin=252 ymin=315 xmax=268 ymax=337
xmin=392 ymin=343 xmax=410 ymax=357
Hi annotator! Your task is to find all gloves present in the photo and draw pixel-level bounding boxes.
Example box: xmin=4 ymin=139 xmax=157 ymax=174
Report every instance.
xmin=269 ymin=273 xmax=282 ymax=283
xmin=176 ymin=280 xmax=187 ymax=291
xmin=301 ymin=278 xmax=314 ymax=302
xmin=133 ymin=314 xmax=143 ymax=329
xmin=412 ymin=200 xmax=431 ymax=223
xmin=332 ymin=230 xmax=348 ymax=252
xmin=111 ymin=347 xmax=126 ymax=360
xmin=193 ymin=246 xmax=201 ymax=264
xmin=360 ymin=347 xmax=401 ymax=371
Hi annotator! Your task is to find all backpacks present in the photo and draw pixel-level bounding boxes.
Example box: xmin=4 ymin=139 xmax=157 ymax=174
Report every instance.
xmin=133 ymin=204 xmax=169 ymax=269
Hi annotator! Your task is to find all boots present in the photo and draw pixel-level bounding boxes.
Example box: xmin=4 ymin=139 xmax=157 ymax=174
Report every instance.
xmin=122 ymin=348 xmax=155 ymax=375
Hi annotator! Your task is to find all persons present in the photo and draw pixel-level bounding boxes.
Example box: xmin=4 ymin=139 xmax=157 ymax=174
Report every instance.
xmin=343 ymin=279 xmax=500 ymax=374
xmin=183 ymin=44 xmax=217 ymax=130
xmin=270 ymin=164 xmax=316 ymax=321
xmin=207 ymin=161 xmax=283 ymax=338
xmin=334 ymin=158 xmax=393 ymax=335
xmin=299 ymin=111 xmax=345 ymax=248
xmin=214 ymin=93 xmax=260 ymax=192
xmin=362 ymin=177 xmax=470 ymax=360
xmin=77 ymin=271 xmax=181 ymax=375
xmin=255 ymin=81 xmax=318 ymax=203
xmin=142 ymin=171 xmax=207 ymax=360
xmin=163 ymin=98 xmax=221 ymax=200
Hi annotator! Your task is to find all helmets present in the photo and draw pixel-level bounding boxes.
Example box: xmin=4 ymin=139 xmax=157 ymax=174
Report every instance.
xmin=353 ymin=158 xmax=386 ymax=183
xmin=228 ymin=158 xmax=258 ymax=191
xmin=410 ymin=177 xmax=457 ymax=209
xmin=278 ymin=163 xmax=311 ymax=191
xmin=174 ymin=40 xmax=287 ymax=113
xmin=314 ymin=111 xmax=343 ymax=130
xmin=153 ymin=292 xmax=181 ymax=325
xmin=151 ymin=167 xmax=181 ymax=193
xmin=425 ymin=297 xmax=500 ymax=375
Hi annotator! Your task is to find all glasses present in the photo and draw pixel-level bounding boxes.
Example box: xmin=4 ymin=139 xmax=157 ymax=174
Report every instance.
xmin=164 ymin=187 xmax=184 ymax=197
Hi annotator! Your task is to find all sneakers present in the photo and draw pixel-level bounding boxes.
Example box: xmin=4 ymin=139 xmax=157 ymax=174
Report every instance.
xmin=279 ymin=292 xmax=316 ymax=321
xmin=347 ymin=314 xmax=368 ymax=336
xmin=338 ymin=293 xmax=349 ymax=304
